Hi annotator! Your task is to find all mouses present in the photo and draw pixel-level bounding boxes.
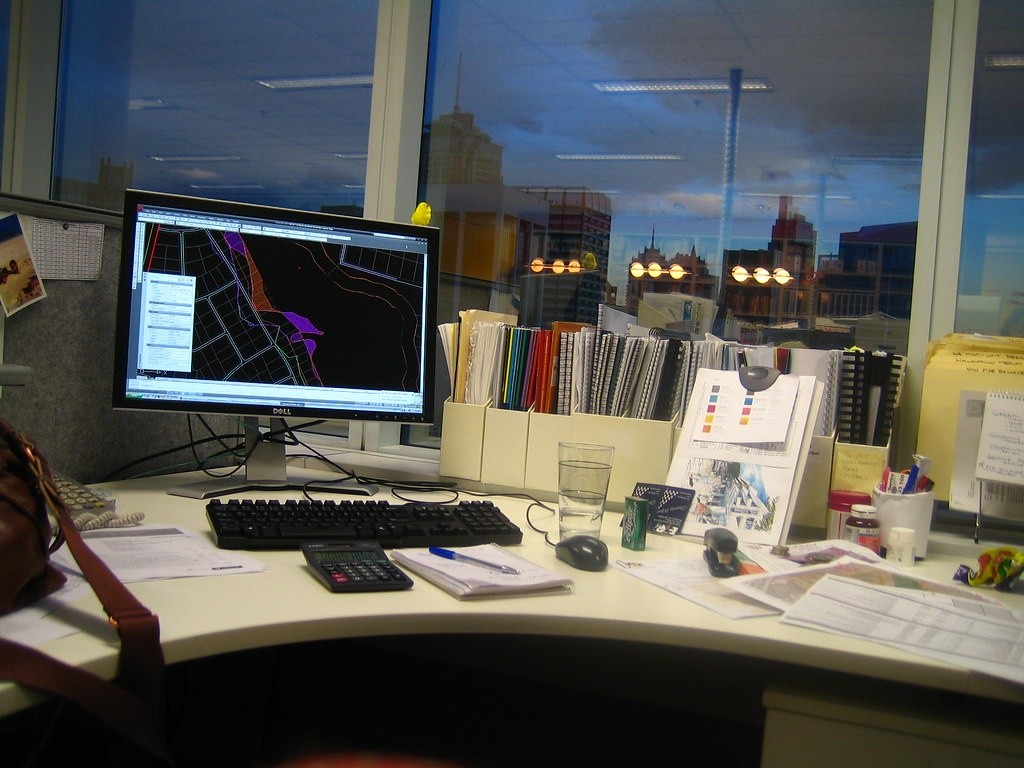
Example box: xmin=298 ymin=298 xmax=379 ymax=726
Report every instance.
xmin=555 ymin=534 xmax=609 ymax=573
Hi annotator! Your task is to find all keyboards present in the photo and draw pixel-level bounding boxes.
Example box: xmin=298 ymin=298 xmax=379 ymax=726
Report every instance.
xmin=203 ymin=497 xmax=523 ymax=550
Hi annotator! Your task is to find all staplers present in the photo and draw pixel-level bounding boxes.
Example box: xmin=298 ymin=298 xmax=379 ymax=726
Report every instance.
xmin=703 ymin=527 xmax=743 ymax=578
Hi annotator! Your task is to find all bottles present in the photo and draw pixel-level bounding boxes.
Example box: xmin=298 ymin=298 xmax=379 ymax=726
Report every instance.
xmin=844 ymin=503 xmax=881 ymax=558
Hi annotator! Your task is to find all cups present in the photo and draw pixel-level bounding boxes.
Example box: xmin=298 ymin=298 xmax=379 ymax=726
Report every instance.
xmin=558 ymin=461 xmax=612 ymax=542
xmin=558 ymin=442 xmax=616 ymax=489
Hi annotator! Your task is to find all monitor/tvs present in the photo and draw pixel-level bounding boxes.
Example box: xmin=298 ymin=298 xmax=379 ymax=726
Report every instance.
xmin=109 ymin=189 xmax=440 ymax=498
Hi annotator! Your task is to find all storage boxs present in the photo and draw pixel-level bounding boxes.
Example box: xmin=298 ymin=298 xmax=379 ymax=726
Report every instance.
xmin=438 ymin=391 xmax=893 ymax=530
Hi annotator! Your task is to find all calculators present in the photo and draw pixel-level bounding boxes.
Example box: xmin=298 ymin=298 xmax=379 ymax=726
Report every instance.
xmin=299 ymin=541 xmax=414 ymax=593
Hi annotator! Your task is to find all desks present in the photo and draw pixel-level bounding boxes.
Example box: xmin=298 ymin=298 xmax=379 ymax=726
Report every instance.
xmin=0 ymin=450 xmax=1024 ymax=725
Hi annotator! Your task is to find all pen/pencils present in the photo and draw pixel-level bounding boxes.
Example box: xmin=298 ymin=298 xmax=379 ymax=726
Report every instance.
xmin=428 ymin=546 xmax=520 ymax=574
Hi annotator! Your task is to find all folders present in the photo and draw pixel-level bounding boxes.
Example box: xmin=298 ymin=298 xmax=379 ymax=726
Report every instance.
xmin=863 ymin=349 xmax=894 ymax=446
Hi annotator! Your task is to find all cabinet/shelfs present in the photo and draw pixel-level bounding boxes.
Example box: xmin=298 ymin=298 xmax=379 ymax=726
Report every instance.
xmin=523 ymin=230 xmax=915 ymax=350
xmin=759 ymin=674 xmax=1024 ymax=768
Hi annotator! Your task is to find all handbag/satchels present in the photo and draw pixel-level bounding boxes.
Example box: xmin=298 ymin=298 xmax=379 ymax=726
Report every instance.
xmin=0 ymin=417 xmax=67 ymax=616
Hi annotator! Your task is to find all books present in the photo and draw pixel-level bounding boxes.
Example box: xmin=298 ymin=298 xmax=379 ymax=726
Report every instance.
xmin=438 ymin=308 xmax=908 ymax=448
xmin=389 ymin=542 xmax=576 ymax=603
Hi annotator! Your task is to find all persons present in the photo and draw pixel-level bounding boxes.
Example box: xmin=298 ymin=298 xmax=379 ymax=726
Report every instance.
xmin=0 ymin=258 xmax=35 ymax=307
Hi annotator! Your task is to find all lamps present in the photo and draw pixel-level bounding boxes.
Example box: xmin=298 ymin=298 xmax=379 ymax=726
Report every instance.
xmin=629 ymin=262 xmax=690 ymax=279
xmin=524 ymin=250 xmax=599 ymax=275
xmin=729 ymin=264 xmax=794 ymax=285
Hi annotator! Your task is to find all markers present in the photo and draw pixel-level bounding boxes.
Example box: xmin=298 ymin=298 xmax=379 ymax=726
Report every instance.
xmin=880 ymin=454 xmax=935 ymax=494
